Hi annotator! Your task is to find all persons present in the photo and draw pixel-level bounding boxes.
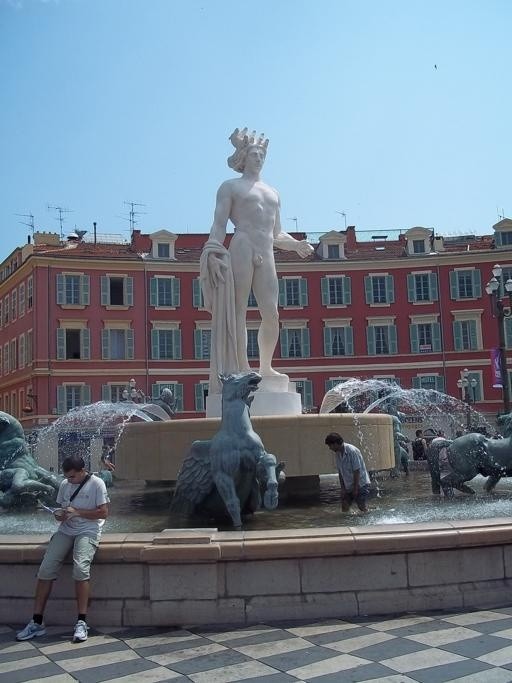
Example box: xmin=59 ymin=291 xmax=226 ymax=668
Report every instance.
xmin=326 ymin=432 xmax=371 ymax=513
xmin=411 ymin=430 xmax=428 ymax=460
xmin=16 ymin=456 xmax=111 ymax=644
xmin=101 ymin=446 xmax=115 ymax=472
xmin=437 ymin=430 xmax=454 ymax=474
xmin=456 ymin=431 xmax=464 ymax=438
xmin=207 ymin=128 xmax=315 ymax=391
xmin=489 ymin=431 xmax=504 ymax=440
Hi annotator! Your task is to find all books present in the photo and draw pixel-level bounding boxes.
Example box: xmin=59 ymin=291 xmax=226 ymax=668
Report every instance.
xmin=41 ymin=502 xmax=80 ymax=520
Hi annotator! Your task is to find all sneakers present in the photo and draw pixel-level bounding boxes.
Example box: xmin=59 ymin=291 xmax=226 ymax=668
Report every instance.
xmin=16 ymin=620 xmax=47 ymax=641
xmin=73 ymin=620 xmax=89 ymax=642
